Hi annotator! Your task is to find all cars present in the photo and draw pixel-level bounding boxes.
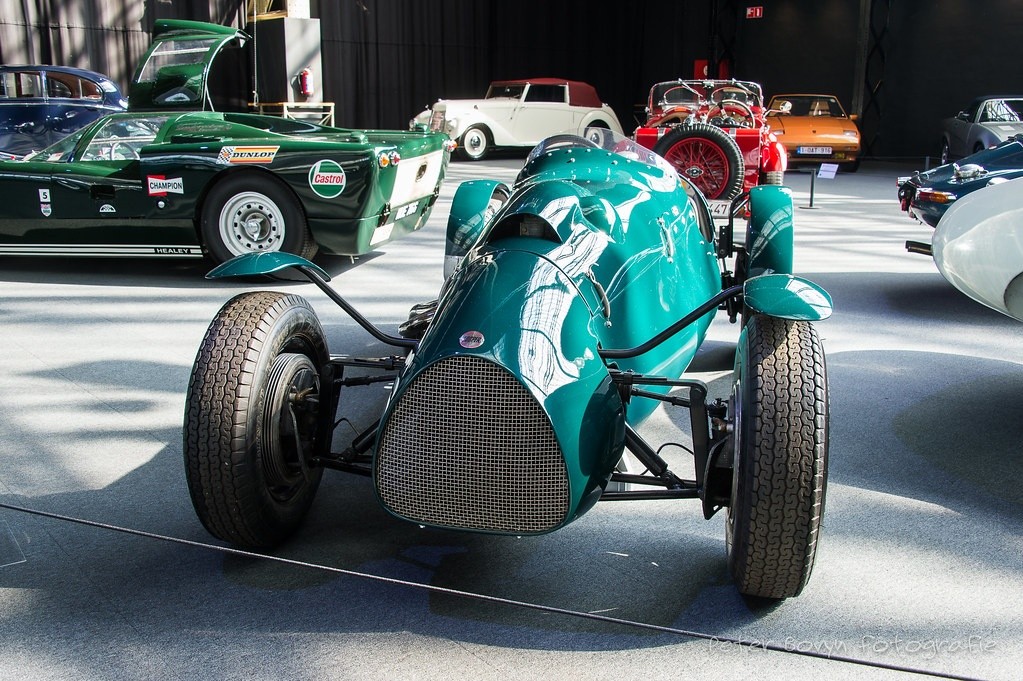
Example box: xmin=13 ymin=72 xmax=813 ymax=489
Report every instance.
xmin=902 ymin=173 xmax=1023 ymax=325
xmin=765 ymin=91 xmax=862 ymax=170
xmin=0 ymin=65 xmax=128 ymax=159
xmin=614 ymin=77 xmax=788 ymax=218
xmin=410 ymin=78 xmax=625 ymax=160
xmin=896 ymin=132 xmax=1023 ymax=229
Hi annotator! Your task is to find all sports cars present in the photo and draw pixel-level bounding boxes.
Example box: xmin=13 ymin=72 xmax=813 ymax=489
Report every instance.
xmin=933 ymin=96 xmax=1022 ymax=163
xmin=0 ymin=17 xmax=456 ymax=285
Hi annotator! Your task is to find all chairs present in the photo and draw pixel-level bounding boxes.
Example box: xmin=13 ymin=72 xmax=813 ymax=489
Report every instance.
xmin=808 ymin=101 xmax=831 ymax=117
xmin=770 ymin=97 xmax=795 ymax=116
